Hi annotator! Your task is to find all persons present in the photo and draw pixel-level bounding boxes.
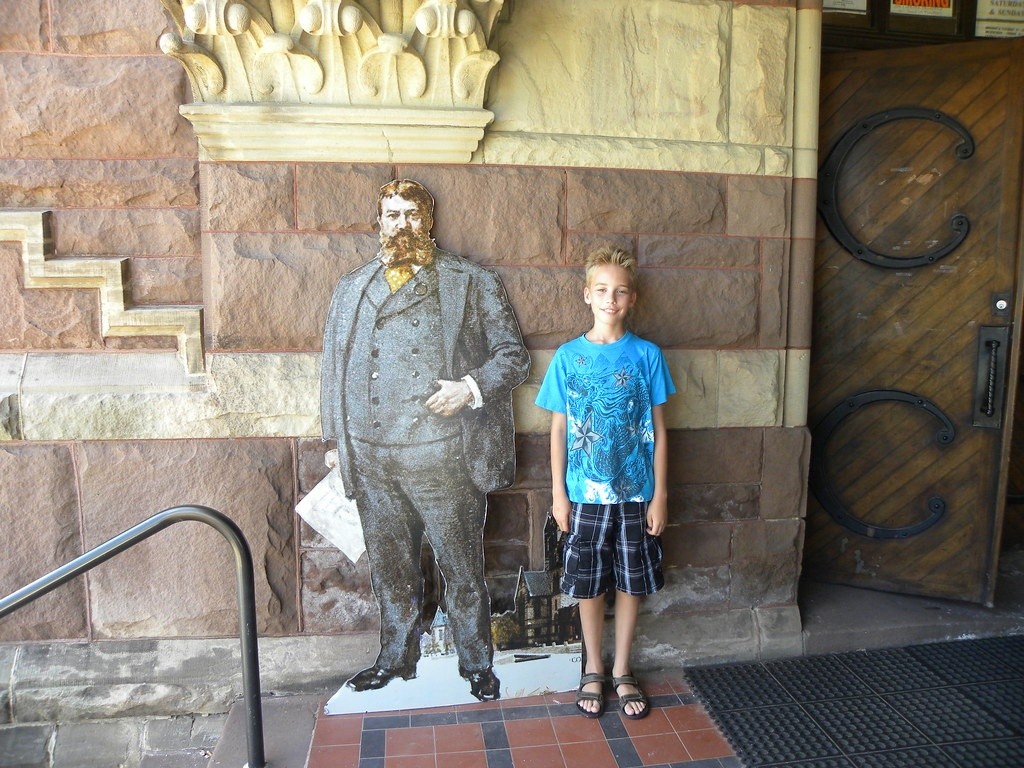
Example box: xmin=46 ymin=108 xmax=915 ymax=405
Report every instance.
xmin=534 ymin=247 xmax=677 ymax=719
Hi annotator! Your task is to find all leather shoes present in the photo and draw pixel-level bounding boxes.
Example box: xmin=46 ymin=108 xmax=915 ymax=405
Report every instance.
xmin=458 ymin=665 xmax=500 ymax=702
xmin=346 ymin=664 xmax=417 ymax=692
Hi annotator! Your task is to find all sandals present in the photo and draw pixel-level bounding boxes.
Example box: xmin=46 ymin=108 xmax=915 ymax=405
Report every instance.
xmin=609 ymin=667 xmax=649 ymax=719
xmin=575 ymin=666 xmax=606 ymax=719
